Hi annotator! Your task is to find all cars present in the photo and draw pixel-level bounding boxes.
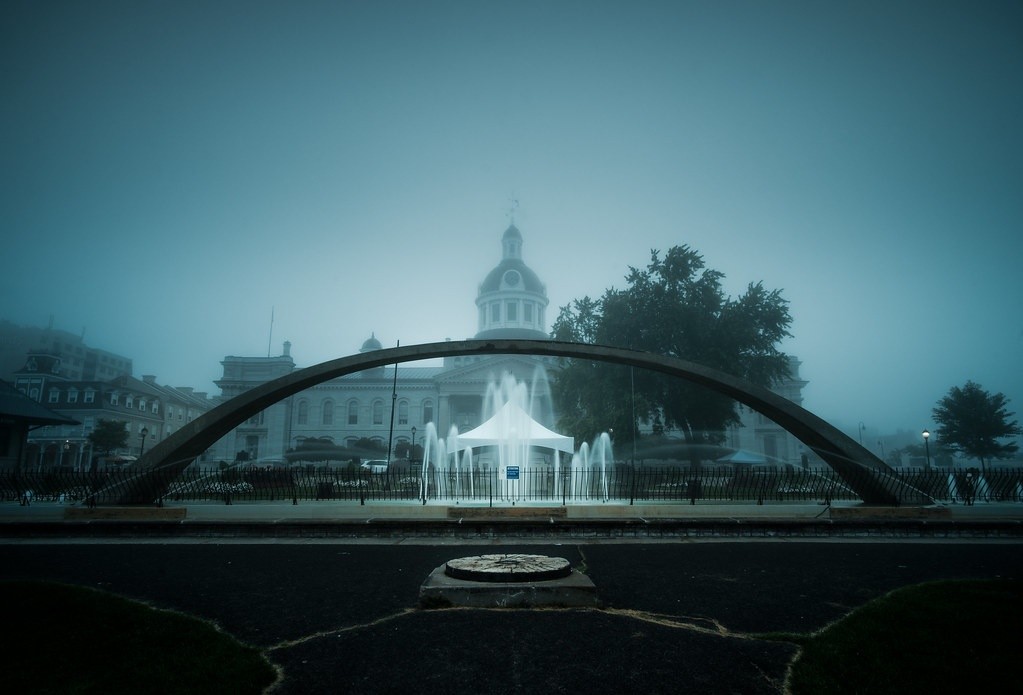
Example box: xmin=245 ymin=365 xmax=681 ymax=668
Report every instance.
xmin=361 ymin=459 xmax=390 ymax=473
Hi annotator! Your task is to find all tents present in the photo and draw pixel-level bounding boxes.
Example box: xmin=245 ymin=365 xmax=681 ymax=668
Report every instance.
xmin=446 ymin=401 xmax=574 ymax=455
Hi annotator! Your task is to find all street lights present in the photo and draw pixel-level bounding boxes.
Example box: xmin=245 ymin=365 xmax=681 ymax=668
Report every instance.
xmin=858 ymin=421 xmax=866 ymax=447
xmin=140 ymin=428 xmax=148 ymax=457
xmin=921 ymin=428 xmax=931 ymax=470
xmin=876 ymin=440 xmax=885 ymax=462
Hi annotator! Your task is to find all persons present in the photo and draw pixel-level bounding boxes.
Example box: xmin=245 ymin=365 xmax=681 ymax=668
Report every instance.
xmin=226 ymin=487 xmax=233 ymax=505
xmin=962 ymin=473 xmax=974 ymax=506
